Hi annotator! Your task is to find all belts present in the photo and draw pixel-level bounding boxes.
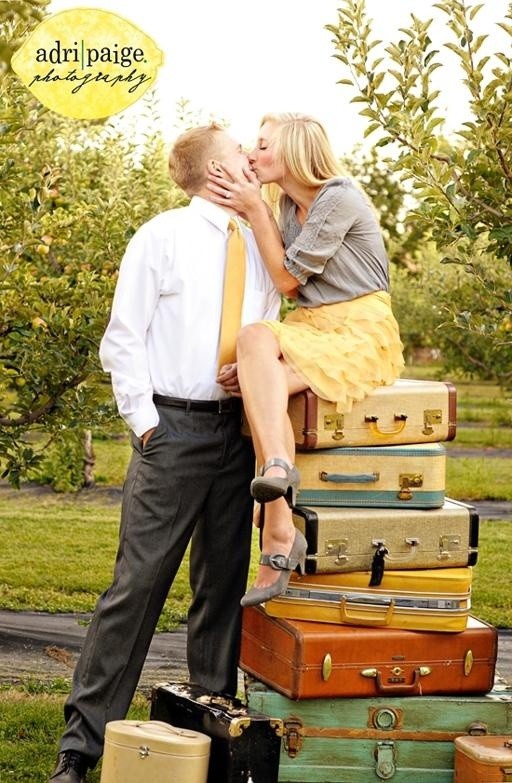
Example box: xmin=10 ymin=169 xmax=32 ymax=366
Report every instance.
xmin=152 ymin=392 xmax=241 ymax=415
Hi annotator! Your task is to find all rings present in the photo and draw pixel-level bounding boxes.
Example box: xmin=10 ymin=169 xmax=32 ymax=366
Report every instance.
xmin=226 ymin=192 xmax=230 ymax=200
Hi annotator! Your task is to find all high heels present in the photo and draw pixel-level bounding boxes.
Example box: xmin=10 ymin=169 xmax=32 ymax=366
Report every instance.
xmin=239 ymin=528 xmax=308 ymax=608
xmin=250 ymin=458 xmax=301 ymax=508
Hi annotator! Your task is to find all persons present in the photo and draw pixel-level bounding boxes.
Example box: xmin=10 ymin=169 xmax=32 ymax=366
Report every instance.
xmin=50 ymin=127 xmax=282 ymax=783
xmin=207 ymin=112 xmax=406 ymax=610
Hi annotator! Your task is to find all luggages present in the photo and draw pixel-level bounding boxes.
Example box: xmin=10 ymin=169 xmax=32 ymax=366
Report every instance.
xmin=243 ymin=669 xmax=512 ymax=783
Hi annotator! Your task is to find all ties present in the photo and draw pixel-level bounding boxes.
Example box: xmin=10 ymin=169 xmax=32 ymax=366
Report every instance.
xmin=217 ymin=219 xmax=249 ymax=373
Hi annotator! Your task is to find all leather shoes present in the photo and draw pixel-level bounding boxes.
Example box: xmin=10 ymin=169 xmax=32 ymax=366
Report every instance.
xmin=46 ymin=751 xmax=90 ymax=783
